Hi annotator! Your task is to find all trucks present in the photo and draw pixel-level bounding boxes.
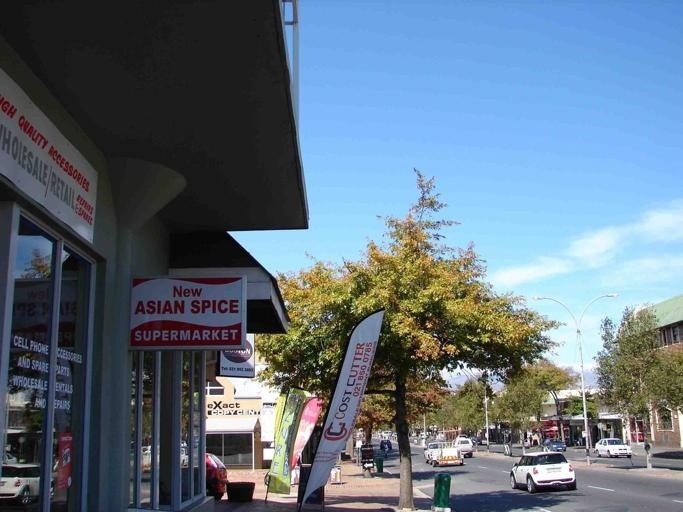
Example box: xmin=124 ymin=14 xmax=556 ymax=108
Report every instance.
xmin=423 ymin=440 xmax=464 ymax=467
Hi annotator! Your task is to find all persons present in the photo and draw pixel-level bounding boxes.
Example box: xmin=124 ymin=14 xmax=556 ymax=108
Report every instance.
xmin=382 ymin=442 xmax=388 ymax=457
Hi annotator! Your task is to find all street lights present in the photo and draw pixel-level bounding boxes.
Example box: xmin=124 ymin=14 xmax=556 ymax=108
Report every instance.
xmin=532 ymin=292 xmax=618 ymax=464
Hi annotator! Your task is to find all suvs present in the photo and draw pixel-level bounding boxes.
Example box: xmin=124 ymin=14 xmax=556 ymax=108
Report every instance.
xmin=0 ymin=462 xmax=55 ymax=505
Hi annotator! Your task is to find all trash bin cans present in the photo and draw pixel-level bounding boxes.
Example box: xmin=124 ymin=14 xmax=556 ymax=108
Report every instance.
xmin=434 ymin=473 xmax=451 ymax=508
xmin=376 ymin=456 xmax=383 ymax=473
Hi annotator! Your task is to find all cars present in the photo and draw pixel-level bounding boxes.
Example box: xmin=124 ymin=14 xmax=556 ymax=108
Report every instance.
xmin=353 ymin=425 xmax=448 ymax=444
xmin=508 ymin=451 xmax=576 ymax=494
xmin=542 ymin=437 xmax=567 ymax=453
xmin=592 ymin=436 xmax=634 ymax=459
xmin=456 ymin=434 xmax=483 ymax=445
xmin=127 ymin=437 xmax=192 ymax=482
xmin=379 ymin=439 xmax=392 ymax=450
xmin=205 ymin=452 xmax=228 ymax=501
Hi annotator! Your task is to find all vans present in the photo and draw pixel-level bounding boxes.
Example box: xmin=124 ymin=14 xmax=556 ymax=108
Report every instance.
xmin=451 ymin=438 xmax=474 ymax=459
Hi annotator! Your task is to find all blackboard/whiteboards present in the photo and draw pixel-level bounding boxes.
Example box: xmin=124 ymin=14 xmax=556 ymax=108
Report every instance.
xmin=297 ymin=464 xmax=324 ymax=504
